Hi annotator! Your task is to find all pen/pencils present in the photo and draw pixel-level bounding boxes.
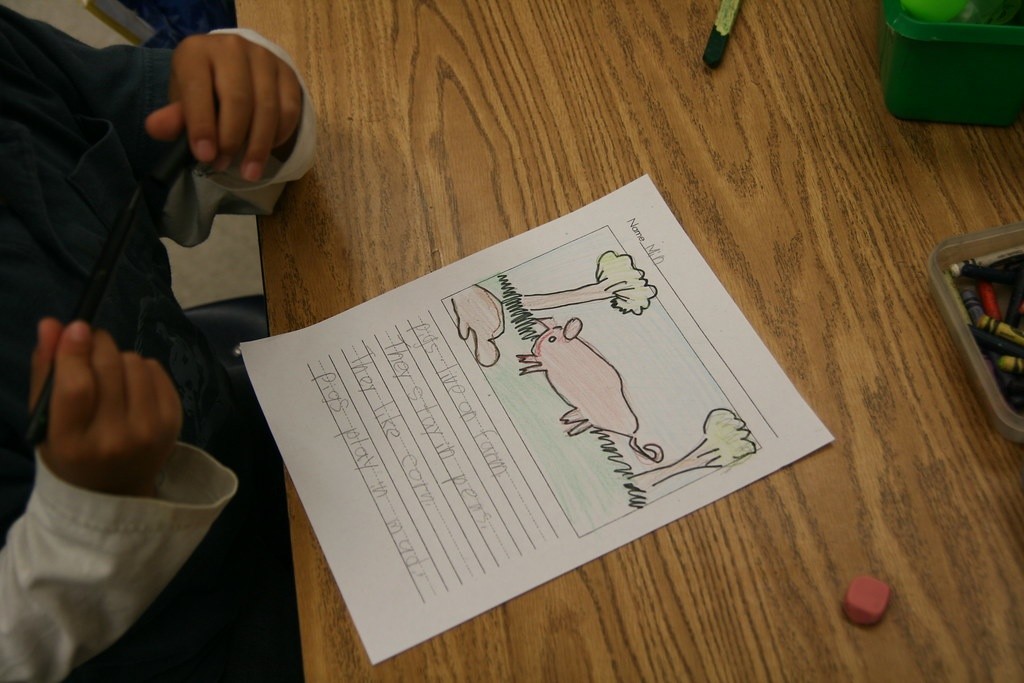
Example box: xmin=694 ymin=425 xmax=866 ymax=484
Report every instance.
xmin=944 ymin=243 xmax=1024 ymax=415
xmin=27 ymin=180 xmax=143 ymax=445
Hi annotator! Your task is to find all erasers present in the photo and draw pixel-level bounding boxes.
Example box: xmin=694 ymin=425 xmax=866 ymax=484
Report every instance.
xmin=846 ymin=570 xmax=891 ymax=623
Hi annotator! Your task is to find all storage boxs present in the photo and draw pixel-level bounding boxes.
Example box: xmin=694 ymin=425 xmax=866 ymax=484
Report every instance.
xmin=873 ymin=0 xmax=1024 ymax=128
xmin=925 ymin=220 xmax=1024 ymax=442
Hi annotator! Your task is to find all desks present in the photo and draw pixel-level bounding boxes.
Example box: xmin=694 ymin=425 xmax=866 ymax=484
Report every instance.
xmin=233 ymin=0 xmax=1024 ymax=683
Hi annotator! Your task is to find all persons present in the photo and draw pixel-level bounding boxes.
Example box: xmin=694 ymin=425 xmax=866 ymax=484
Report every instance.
xmin=0 ymin=5 xmax=318 ymax=683
